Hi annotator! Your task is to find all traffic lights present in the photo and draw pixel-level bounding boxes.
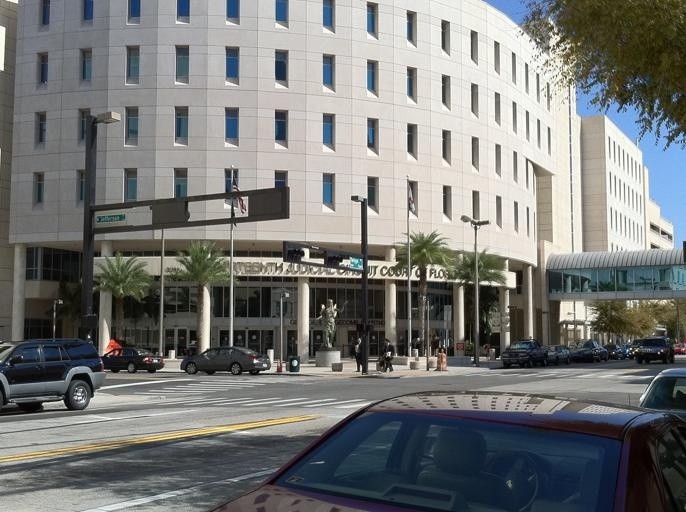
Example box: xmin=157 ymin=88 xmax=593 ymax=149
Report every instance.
xmin=287 ymin=244 xmax=305 ymax=262
xmin=325 ymin=252 xmax=343 ymax=266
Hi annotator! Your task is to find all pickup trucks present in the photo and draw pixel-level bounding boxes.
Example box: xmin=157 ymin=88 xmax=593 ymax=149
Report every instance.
xmin=501 ymin=339 xmax=549 ymax=367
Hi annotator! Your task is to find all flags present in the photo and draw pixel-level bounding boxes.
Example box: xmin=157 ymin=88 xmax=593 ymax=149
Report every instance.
xmin=233 ymin=176 xmax=246 ymax=216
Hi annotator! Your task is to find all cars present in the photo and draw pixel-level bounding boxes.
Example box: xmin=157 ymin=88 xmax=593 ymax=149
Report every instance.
xmin=548 ymin=336 xmax=686 ymax=366
xmin=102 ymin=347 xmax=165 ymax=374
xmin=208 ymin=388 xmax=686 ymax=511
xmin=180 ymin=346 xmax=272 ymax=375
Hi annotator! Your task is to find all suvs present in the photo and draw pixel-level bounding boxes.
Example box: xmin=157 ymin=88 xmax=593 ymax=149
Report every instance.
xmin=0 ymin=337 xmax=106 ymax=411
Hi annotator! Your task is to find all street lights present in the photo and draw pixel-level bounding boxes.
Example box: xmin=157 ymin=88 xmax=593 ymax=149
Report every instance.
xmin=83 ymin=110 xmax=122 ymax=353
xmin=279 ymin=292 xmax=289 ymax=368
xmin=350 ymin=194 xmax=371 ymax=378
xmin=460 ymin=215 xmax=490 ymax=364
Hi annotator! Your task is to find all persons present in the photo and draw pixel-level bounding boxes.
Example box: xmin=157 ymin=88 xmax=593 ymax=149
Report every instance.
xmin=416 ymin=337 xmax=423 ymax=356
xmin=382 ymin=339 xmax=395 ymax=372
xmin=316 ymin=299 xmax=338 ymax=348
xmin=353 ymin=337 xmax=362 ymax=372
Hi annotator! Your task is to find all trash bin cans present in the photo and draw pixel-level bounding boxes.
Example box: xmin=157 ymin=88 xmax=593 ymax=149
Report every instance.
xmin=290 ymin=356 xmax=300 ymax=373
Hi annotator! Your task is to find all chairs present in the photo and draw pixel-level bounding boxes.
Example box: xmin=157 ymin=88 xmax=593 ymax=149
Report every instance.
xmin=355 ymin=425 xmax=601 ymax=512
